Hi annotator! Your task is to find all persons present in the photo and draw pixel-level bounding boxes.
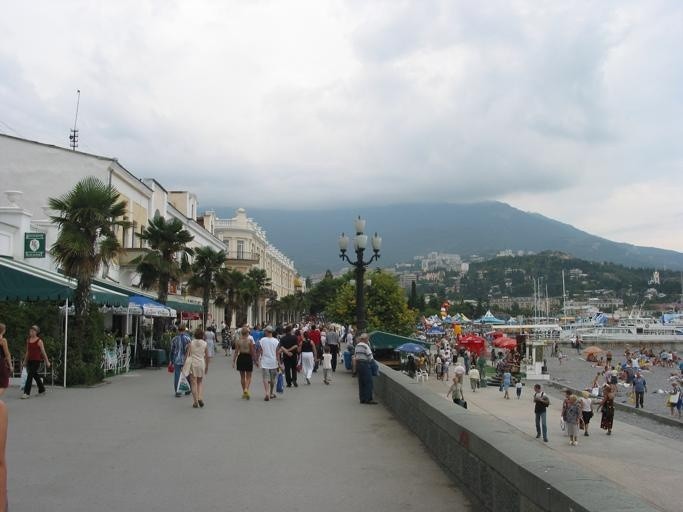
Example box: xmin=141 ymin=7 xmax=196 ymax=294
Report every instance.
xmin=394 ymin=324 xmax=683 ymax=447
xmin=21 ymin=326 xmax=52 ymax=399
xmin=167 ymin=316 xmax=379 ymax=409
xmin=0 ymin=399 xmax=9 ymax=512
xmin=0 ymin=323 xmax=14 ymax=398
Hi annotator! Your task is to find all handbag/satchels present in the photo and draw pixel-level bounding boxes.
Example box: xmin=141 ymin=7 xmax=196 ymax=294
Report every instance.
xmin=182 ymin=356 xmax=191 ymax=377
xmin=277 ymin=368 xmax=284 ymax=393
xmin=296 ymin=362 xmax=302 ymax=370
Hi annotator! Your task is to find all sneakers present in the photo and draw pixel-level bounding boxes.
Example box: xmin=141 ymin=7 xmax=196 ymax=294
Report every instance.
xmin=198 ymin=399 xmax=204 ymax=407
xmin=21 ymin=394 xmax=29 ymax=398
xmin=242 ymin=388 xmax=250 ymax=399
xmin=193 ymin=403 xmax=198 ymax=408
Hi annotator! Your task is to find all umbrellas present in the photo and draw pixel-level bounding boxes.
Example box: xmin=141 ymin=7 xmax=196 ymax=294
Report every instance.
xmin=394 ymin=310 xmax=517 ymax=353
xmin=98 ymin=293 xmax=177 ymax=376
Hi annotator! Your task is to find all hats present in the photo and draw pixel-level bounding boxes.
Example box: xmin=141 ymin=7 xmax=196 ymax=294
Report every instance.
xmin=263 ymin=326 xmax=273 ymax=332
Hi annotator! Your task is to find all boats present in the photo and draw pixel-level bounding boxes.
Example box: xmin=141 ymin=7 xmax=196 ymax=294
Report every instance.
xmin=571 ymin=295 xmax=683 ymax=343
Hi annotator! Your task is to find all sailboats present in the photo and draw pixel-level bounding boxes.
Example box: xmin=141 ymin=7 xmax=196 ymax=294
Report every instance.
xmin=487 ymin=270 xmax=574 ymax=344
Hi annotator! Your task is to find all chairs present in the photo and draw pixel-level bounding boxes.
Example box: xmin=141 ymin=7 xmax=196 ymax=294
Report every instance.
xmin=92 ymin=339 xmax=131 ymax=375
xmin=400 ymin=367 xmax=429 ymax=382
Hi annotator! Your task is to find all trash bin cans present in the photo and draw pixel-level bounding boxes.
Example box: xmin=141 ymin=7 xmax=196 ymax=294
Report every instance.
xmin=343 ymin=351 xmax=352 ymax=369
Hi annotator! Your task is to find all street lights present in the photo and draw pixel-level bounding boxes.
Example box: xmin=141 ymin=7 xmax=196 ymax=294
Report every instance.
xmin=336 ymin=215 xmax=382 ymax=333
